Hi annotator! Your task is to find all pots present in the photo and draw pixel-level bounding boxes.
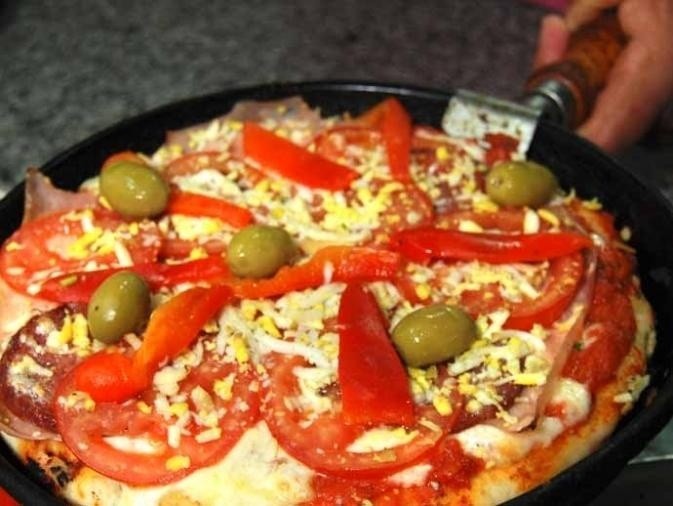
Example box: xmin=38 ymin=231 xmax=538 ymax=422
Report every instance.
xmin=0 ymin=13 xmax=671 ymax=506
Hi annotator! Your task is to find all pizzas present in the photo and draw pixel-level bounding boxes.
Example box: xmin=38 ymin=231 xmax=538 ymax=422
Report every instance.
xmin=0 ymin=96 xmax=657 ymax=506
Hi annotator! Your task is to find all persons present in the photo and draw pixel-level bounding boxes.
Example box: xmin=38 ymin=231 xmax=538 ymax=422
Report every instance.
xmin=534 ymin=1 xmax=673 ymax=146
